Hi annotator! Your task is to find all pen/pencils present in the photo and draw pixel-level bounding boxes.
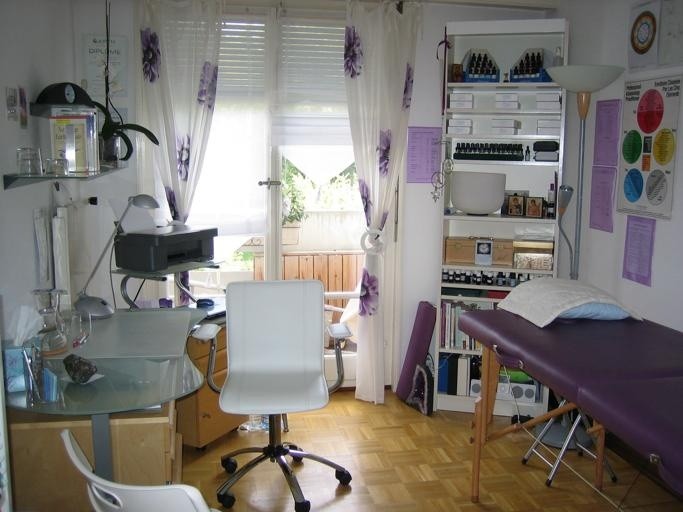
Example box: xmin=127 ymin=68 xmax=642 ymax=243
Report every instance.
xmin=23 ymin=343 xmax=41 ymax=406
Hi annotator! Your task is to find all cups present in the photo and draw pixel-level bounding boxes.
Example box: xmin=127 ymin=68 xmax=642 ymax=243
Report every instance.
xmin=45 ymin=158 xmax=69 ymax=175
xmin=23 ymin=359 xmax=42 ymax=403
xmin=17 ymin=147 xmax=43 ymax=176
xmin=70 ymin=312 xmax=92 ymax=343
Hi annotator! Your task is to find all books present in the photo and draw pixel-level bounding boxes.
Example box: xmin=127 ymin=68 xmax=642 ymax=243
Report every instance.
xmin=441 ymin=302 xmax=481 ymax=351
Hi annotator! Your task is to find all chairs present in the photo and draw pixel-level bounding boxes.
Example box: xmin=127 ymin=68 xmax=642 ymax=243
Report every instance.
xmin=60 ymin=429 xmax=221 ymax=512
xmin=191 ymin=280 xmax=353 ymax=512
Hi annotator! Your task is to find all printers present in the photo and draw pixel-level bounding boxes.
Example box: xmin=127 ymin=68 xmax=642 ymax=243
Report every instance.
xmin=114 ymin=220 xmax=218 ymax=273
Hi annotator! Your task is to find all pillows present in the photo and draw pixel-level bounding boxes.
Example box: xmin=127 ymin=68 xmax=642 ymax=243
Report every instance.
xmin=559 ymin=303 xmax=631 ymax=320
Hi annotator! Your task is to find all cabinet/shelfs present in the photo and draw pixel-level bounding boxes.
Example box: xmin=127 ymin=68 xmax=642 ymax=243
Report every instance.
xmin=432 ymin=18 xmax=570 ymax=419
xmin=175 ymin=320 xmax=249 ymax=451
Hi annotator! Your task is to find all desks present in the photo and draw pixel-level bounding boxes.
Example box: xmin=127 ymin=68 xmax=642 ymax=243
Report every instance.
xmin=8 ymin=310 xmax=209 ymax=512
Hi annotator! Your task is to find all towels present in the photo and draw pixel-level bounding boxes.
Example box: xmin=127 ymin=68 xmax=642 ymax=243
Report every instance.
xmin=497 ymin=278 xmax=644 ymax=328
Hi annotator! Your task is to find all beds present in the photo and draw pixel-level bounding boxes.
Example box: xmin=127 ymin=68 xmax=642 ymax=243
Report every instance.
xmin=457 ymin=311 xmax=683 ymax=512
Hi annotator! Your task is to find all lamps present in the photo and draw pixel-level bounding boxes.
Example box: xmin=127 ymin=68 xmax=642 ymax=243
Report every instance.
xmin=523 ymin=65 xmax=626 ymax=487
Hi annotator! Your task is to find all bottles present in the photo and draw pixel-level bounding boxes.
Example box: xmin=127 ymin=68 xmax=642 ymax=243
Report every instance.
xmin=547 ymin=183 xmax=556 ymax=218
xmin=442 ymin=269 xmax=529 ymax=287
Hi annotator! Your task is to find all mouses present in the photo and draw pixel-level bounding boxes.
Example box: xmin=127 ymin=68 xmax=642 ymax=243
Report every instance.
xmin=197 ymin=299 xmax=214 ymax=306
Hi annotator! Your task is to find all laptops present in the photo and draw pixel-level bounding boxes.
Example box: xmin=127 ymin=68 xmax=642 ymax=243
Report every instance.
xmin=180 ymin=297 xmax=226 ymax=320
